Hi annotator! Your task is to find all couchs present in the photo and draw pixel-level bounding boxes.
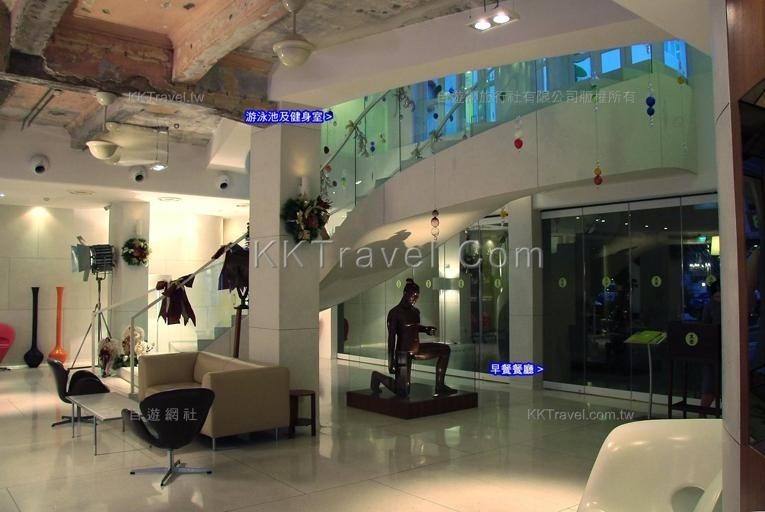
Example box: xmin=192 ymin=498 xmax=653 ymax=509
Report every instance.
xmin=136 ymin=350 xmax=292 ymax=456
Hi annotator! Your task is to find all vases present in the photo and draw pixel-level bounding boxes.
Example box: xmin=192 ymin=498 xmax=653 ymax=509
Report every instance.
xmin=47 ymin=285 xmax=67 ymax=364
xmin=23 ymin=285 xmax=44 ymax=369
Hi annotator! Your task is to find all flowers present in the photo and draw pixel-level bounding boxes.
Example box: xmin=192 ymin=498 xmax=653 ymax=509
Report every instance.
xmin=121 ymin=237 xmax=152 ymax=267
xmin=95 ymin=324 xmax=155 ymax=379
xmin=281 ymin=192 xmax=333 ymax=244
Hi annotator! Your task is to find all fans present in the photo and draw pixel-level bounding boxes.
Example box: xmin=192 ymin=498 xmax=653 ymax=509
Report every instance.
xmin=24 ymin=91 xmax=174 ymax=166
xmin=236 ymin=0 xmax=379 ymax=73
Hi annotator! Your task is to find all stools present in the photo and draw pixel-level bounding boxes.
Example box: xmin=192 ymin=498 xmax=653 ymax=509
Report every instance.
xmin=288 ymin=389 xmax=316 ymax=440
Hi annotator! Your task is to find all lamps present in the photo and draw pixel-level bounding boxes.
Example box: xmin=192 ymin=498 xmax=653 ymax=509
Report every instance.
xmin=465 ymin=0 xmax=522 ymax=33
xmin=69 ymin=234 xmax=118 ymax=372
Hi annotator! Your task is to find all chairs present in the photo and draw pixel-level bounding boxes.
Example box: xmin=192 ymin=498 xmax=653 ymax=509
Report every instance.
xmin=573 ymin=417 xmax=725 ymax=511
xmin=0 ymin=322 xmax=15 ymax=369
xmin=46 ymin=356 xmax=109 ymax=428
xmin=121 ymin=387 xmax=218 ymax=490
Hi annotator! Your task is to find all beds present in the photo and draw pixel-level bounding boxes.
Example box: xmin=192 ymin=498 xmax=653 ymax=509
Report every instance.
xmin=61 ymin=391 xmax=140 ymax=459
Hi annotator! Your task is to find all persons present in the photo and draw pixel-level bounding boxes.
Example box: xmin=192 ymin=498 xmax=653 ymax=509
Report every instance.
xmin=699 ymin=282 xmax=721 ymax=418
xmin=370 ymin=278 xmax=458 ymax=396
